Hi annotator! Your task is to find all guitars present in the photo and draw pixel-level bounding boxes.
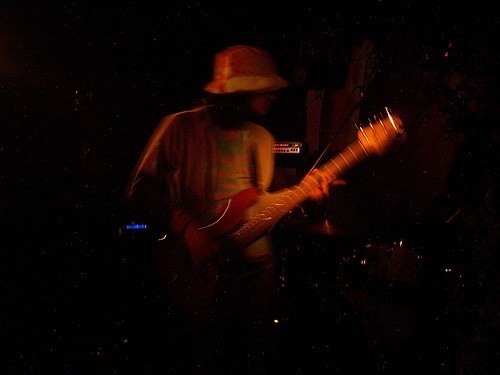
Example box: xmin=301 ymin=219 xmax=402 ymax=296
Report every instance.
xmin=115 ymin=108 xmax=407 ymax=308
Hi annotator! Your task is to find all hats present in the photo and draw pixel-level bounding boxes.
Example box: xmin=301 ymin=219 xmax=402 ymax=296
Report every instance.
xmin=203 ymin=45 xmax=290 ymax=93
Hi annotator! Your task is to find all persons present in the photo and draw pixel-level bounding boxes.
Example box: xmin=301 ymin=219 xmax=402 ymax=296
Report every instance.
xmin=129 ymin=44 xmax=344 ymax=322
